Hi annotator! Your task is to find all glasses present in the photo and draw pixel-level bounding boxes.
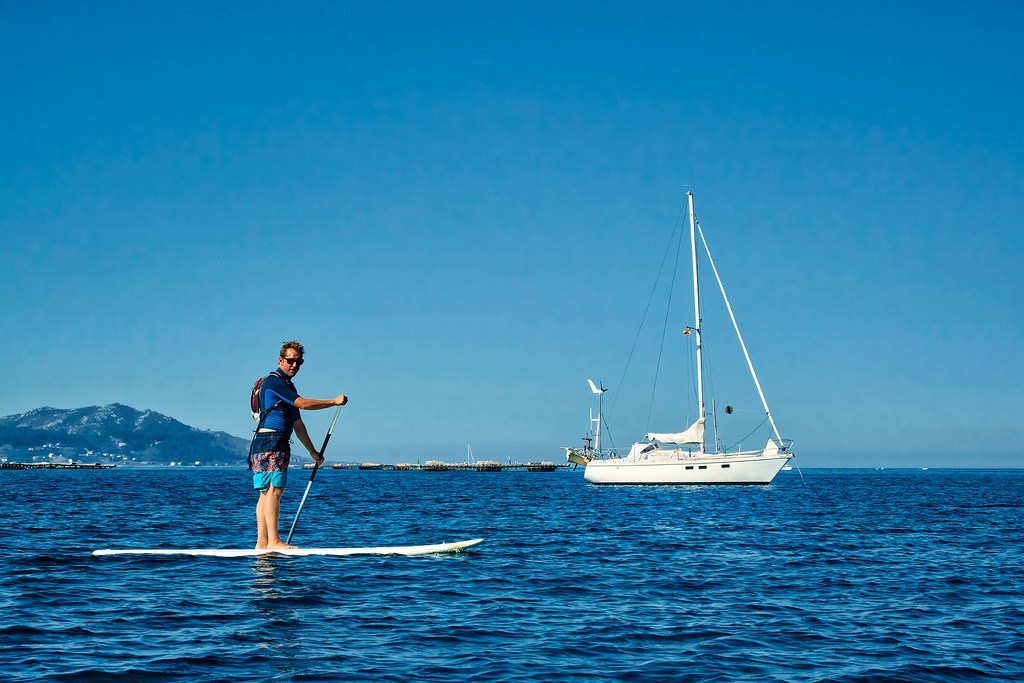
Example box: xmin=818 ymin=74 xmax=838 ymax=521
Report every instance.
xmin=281 ymin=356 xmax=304 ymax=365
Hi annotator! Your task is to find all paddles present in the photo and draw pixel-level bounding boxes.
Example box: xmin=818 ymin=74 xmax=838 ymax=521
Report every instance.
xmin=285 ymin=402 xmax=343 ymax=544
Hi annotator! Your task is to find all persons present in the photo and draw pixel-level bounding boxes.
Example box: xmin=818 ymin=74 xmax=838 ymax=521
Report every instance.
xmin=248 ymin=341 xmax=348 ymax=549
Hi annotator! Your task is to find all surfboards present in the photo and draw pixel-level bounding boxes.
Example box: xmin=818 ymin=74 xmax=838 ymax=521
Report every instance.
xmin=94 ymin=536 xmax=483 ymax=559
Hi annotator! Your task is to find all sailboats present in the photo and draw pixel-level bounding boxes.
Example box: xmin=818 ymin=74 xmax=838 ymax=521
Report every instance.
xmin=560 ymin=183 xmax=805 ymax=486
